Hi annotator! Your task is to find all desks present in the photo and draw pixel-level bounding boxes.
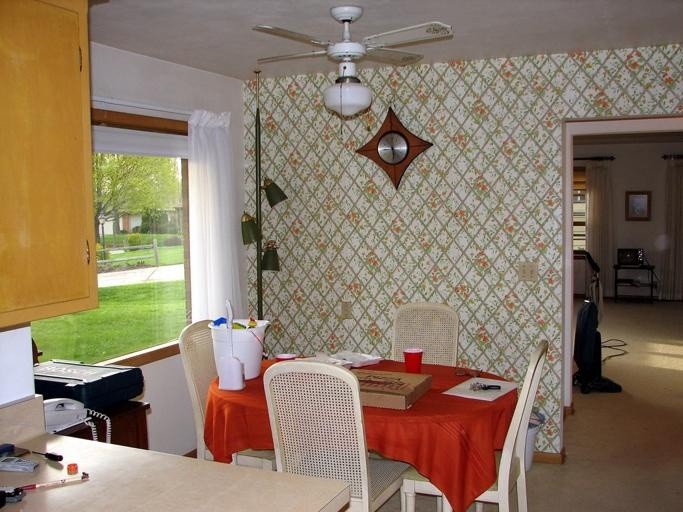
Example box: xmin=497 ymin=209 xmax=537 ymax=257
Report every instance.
xmin=205 ymin=355 xmax=519 ymax=511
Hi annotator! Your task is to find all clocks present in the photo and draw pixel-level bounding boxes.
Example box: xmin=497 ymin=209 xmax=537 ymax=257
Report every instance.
xmin=354 ymin=107 xmax=433 ymax=191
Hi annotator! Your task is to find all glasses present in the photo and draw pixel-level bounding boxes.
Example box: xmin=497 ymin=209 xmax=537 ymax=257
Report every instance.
xmin=455 ymin=370 xmax=481 ymax=377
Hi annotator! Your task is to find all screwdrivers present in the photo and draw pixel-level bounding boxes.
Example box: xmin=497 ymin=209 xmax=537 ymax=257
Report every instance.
xmin=33 ymin=450 xmax=63 ymax=461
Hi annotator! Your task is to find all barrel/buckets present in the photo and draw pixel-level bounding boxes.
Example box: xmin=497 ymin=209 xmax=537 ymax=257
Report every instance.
xmin=208 ymin=319 xmax=271 ymax=381
xmin=524 ymin=426 xmax=542 ymax=473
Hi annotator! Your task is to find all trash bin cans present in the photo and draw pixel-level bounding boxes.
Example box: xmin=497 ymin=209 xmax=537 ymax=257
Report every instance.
xmin=525 ymin=423 xmax=541 ymax=472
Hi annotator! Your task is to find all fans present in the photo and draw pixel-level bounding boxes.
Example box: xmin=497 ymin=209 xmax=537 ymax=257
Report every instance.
xmin=252 ymin=3 xmax=453 ymax=64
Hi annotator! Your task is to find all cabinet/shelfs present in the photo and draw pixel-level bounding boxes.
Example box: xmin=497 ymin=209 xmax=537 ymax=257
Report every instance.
xmin=1 ymin=0 xmax=96 ymax=329
xmin=613 ymin=265 xmax=655 ymax=302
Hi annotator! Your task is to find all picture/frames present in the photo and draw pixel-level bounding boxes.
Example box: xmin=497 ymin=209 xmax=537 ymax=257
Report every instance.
xmin=625 ymin=191 xmax=652 ymax=222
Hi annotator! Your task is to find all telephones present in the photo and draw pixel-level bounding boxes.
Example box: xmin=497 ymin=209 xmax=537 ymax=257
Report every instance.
xmin=43 ymin=398 xmax=87 ymax=426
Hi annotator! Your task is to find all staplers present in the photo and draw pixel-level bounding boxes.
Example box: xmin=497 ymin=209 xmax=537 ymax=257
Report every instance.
xmin=0 ymin=444 xmax=15 ymax=456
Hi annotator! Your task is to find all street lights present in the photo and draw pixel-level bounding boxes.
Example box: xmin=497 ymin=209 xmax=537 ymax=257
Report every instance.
xmin=98 ymin=208 xmax=108 ymax=268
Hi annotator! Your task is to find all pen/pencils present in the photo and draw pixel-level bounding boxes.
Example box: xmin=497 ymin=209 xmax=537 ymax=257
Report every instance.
xmin=18 ymin=472 xmax=90 ymax=490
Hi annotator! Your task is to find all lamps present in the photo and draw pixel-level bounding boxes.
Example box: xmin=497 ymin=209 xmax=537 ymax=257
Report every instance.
xmin=239 ymin=68 xmax=287 ymax=319
xmin=323 ymin=61 xmax=372 ymax=118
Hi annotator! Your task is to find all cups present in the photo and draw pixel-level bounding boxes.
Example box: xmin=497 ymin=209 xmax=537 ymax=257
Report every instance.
xmin=274 ymin=353 xmax=297 ymax=362
xmin=402 ymin=348 xmax=424 ymax=375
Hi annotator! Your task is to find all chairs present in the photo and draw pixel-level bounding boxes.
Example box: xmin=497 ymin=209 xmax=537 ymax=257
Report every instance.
xmin=261 ymin=359 xmax=410 ymax=512
xmin=397 ymin=338 xmax=549 ymax=512
xmin=389 ymin=301 xmax=461 ymax=368
xmin=179 ymin=316 xmax=277 ymax=472
